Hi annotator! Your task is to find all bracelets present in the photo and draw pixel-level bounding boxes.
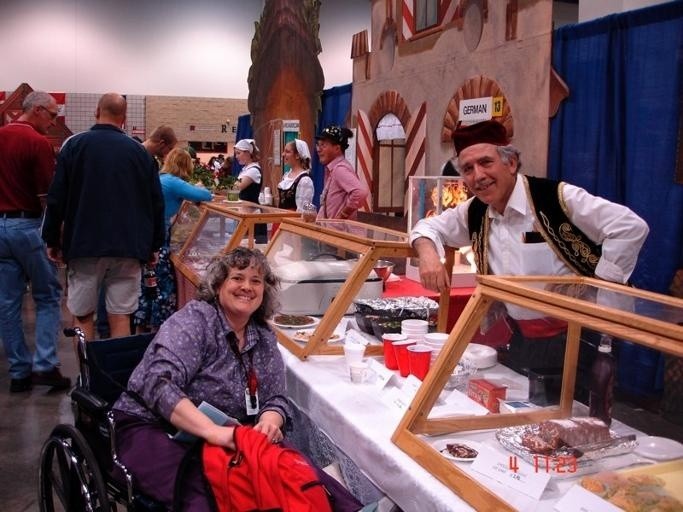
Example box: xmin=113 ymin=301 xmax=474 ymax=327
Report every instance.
xmin=340 ymin=210 xmax=348 ymax=218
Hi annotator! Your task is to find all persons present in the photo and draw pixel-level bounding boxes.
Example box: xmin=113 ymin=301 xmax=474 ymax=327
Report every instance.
xmin=404 ymin=118 xmax=651 ymax=409
xmin=0 ymin=90 xmax=72 ymax=394
xmin=40 ymin=92 xmax=166 ymax=374
xmin=139 ymin=124 xmax=176 ymax=170
xmin=313 ymin=125 xmax=369 ymax=263
xmin=106 ymin=243 xmax=294 ymax=511
xmin=129 ymin=147 xmax=212 ymax=337
xmin=229 ymin=138 xmax=268 ymax=243
xmin=270 ymin=138 xmax=314 ymax=240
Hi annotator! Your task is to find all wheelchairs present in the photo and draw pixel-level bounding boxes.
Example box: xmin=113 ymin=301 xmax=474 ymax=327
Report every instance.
xmin=37 ymin=326 xmax=166 ymax=511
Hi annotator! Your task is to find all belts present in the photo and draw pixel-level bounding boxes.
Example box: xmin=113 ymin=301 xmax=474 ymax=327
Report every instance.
xmin=0 ymin=210 xmax=43 ymax=220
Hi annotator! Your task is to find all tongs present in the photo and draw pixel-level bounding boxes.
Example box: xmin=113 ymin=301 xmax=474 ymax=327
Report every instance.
xmin=557 ymin=432 xmax=635 ymax=457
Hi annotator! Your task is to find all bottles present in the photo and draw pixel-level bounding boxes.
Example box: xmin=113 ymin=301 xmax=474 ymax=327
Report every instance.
xmin=589 ymin=333 xmax=614 ymax=429
xmin=303 ymin=202 xmax=318 ymax=224
xmin=143 ymin=263 xmax=157 ymax=299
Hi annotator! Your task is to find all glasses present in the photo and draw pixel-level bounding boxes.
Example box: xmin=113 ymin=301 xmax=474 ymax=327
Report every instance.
xmin=41 ymin=105 xmax=57 ymax=120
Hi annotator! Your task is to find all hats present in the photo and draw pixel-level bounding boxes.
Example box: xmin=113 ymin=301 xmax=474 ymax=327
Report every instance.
xmin=313 ymin=125 xmax=349 ymax=149
xmin=452 ymin=120 xmax=509 ymax=156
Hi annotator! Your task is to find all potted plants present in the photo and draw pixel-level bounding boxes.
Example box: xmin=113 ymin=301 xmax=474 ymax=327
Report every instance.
xmin=222 ymin=175 xmax=242 ymax=201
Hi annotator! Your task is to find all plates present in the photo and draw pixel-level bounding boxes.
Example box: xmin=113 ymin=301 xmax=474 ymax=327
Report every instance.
xmin=223 ymin=200 xmax=244 ymax=204
xmin=461 ymin=340 xmax=501 ymax=370
xmin=633 ymin=434 xmax=682 ymax=462
xmin=271 ymin=311 xmax=345 ymax=344
xmin=432 ymin=440 xmax=486 ymax=461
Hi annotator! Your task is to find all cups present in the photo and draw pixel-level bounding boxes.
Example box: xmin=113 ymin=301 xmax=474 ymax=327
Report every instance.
xmin=381 ymin=316 xmax=459 ymax=382
xmin=258 ymin=186 xmax=272 ymax=205
xmin=226 ymin=190 xmax=240 ymax=200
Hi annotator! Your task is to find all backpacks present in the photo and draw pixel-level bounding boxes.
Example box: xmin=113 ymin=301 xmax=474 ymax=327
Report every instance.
xmin=170 ymin=422 xmax=335 ymax=512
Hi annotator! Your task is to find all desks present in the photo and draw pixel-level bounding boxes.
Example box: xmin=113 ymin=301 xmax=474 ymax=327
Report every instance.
xmin=382 ymin=274 xmax=513 ymax=348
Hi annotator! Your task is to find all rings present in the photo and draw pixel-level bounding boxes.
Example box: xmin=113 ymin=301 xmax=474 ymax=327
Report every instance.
xmin=271 ymin=438 xmax=277 ymax=443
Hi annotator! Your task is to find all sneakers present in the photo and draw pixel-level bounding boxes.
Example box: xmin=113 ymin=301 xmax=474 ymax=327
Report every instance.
xmin=31 ymin=366 xmax=72 ymax=387
xmin=358 ymin=496 xmax=398 ymax=512
xmin=10 ymin=378 xmax=33 ymax=392
